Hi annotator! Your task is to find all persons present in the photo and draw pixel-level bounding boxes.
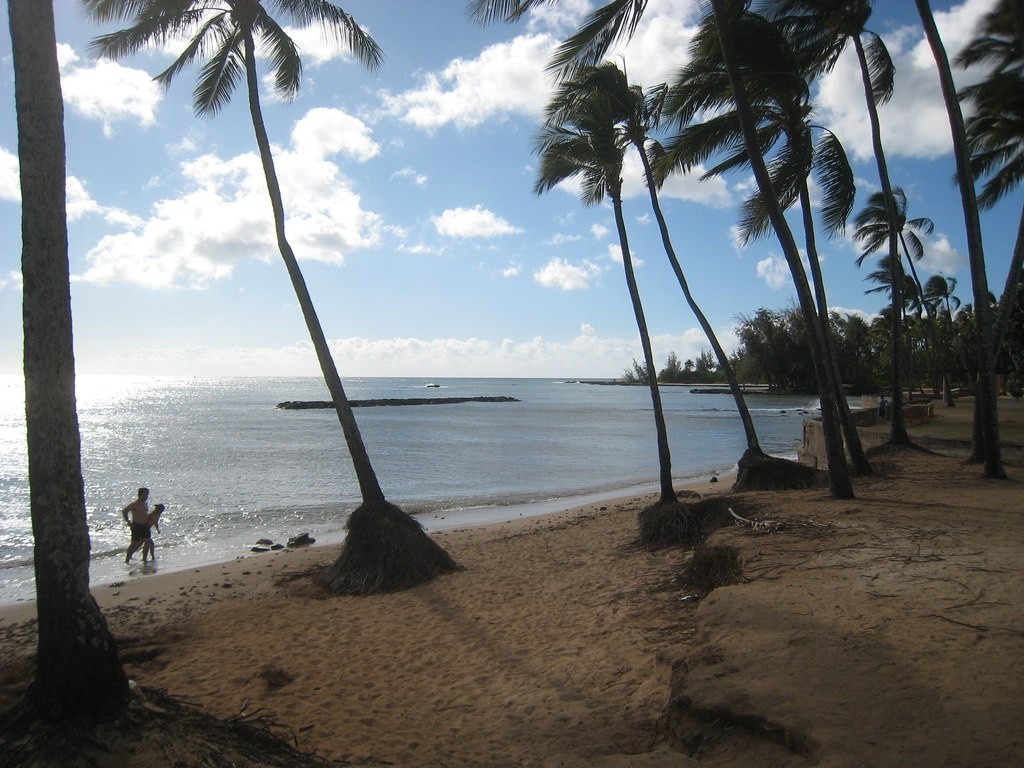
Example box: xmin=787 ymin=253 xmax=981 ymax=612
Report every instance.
xmin=877 ymin=396 xmax=887 ymax=423
xmin=122 ymin=488 xmax=151 ymax=565
xmin=130 ymin=504 xmax=164 ymax=561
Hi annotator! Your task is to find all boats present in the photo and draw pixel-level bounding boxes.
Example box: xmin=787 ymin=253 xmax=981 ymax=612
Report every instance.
xmin=427 ymin=384 xmax=440 ymax=388
xmin=564 ymin=380 xmax=577 ymax=383
